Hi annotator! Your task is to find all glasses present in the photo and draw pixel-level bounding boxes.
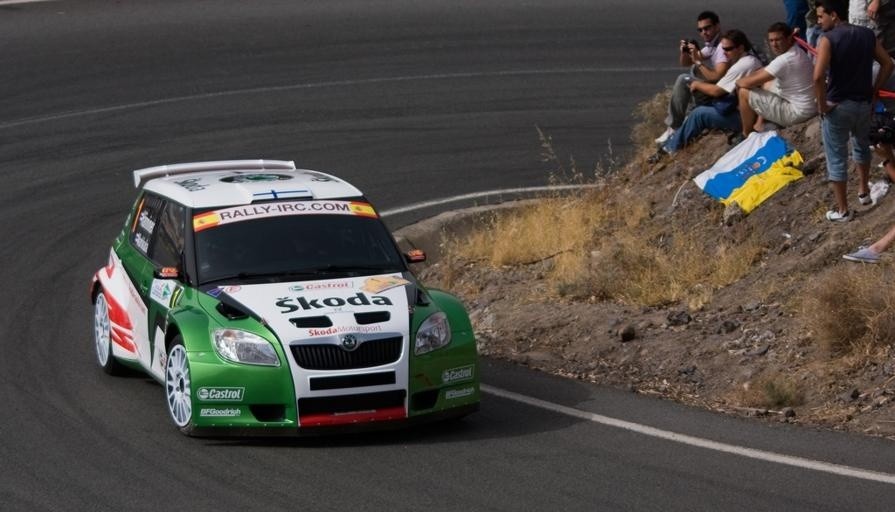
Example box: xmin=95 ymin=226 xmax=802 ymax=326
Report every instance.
xmin=696 ymin=24 xmax=714 ymax=33
xmin=722 ymin=46 xmax=737 ymax=52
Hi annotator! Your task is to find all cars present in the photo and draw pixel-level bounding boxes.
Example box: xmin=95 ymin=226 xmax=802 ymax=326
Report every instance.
xmin=91 ymin=158 xmax=483 ymax=439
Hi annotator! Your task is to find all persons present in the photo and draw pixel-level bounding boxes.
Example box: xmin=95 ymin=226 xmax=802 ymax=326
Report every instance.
xmin=645 ymin=0 xmax=895 ymax=222
xmin=842 ymin=226 xmax=895 ymax=263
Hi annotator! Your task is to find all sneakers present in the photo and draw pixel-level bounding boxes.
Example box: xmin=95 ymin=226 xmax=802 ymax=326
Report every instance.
xmin=858 ymin=182 xmax=874 ymax=205
xmin=647 ymin=147 xmax=669 ymax=163
xmin=843 ymin=246 xmax=880 ymax=264
xmin=654 ymin=126 xmax=678 ymax=144
xmin=825 ymin=207 xmax=855 ymax=222
xmin=731 ymin=127 xmax=758 ymax=145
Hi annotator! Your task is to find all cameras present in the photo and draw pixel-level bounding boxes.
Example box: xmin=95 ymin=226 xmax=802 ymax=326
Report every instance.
xmin=682 ymin=39 xmax=700 ymax=53
